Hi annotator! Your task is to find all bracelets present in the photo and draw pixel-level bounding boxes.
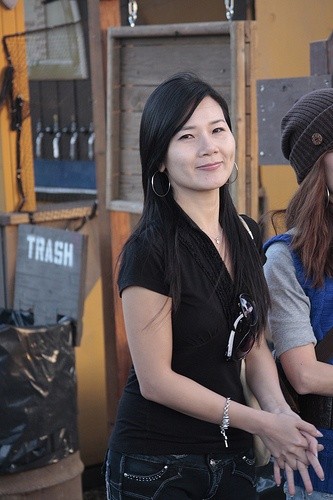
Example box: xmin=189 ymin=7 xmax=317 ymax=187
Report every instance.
xmin=219 ymin=396 xmax=231 ymax=448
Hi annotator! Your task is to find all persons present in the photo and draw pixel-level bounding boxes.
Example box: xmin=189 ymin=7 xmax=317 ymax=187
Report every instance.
xmin=103 ymin=71 xmax=328 ymax=500
xmin=263 ymin=87 xmax=332 ymax=496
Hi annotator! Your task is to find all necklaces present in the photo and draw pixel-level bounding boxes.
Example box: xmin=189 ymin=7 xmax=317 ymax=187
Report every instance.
xmin=205 ymin=228 xmax=224 ymax=245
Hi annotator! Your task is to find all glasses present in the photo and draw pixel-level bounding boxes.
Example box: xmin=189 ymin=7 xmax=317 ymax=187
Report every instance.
xmin=225 ymin=292 xmax=258 ymax=362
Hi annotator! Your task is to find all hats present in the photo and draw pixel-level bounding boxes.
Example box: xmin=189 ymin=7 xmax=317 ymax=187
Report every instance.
xmin=280 ymin=87 xmax=333 ymax=184
